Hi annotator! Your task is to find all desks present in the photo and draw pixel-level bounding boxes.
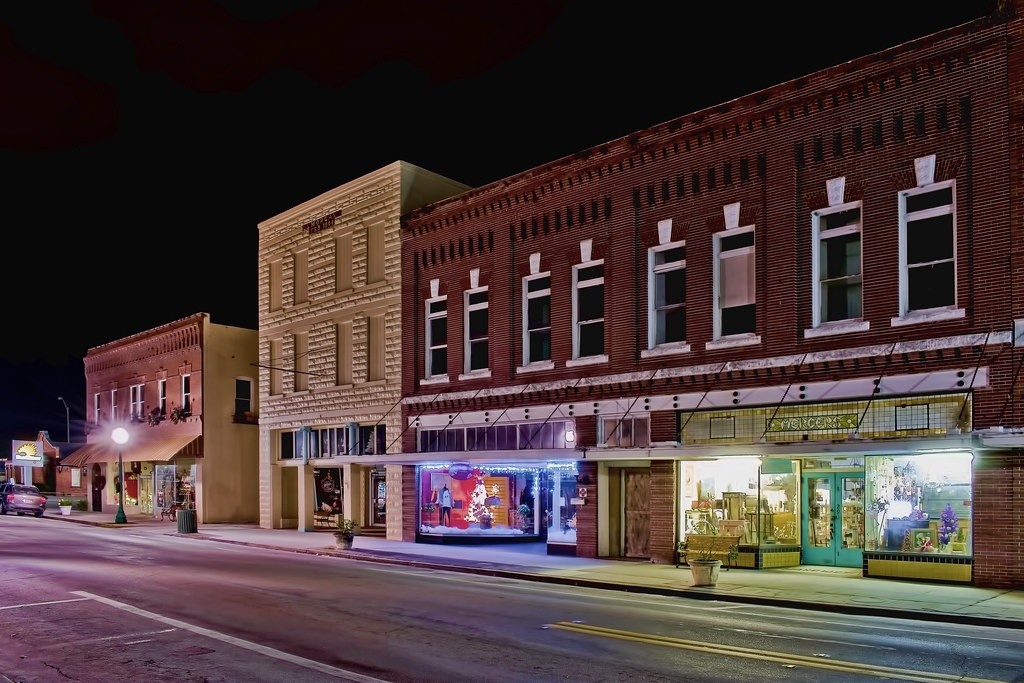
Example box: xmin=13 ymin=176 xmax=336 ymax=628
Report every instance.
xmin=722 ymin=492 xmax=746 ymax=520
xmin=887 ymin=519 xmax=930 ymax=550
xmin=719 ymin=520 xmax=749 ymax=544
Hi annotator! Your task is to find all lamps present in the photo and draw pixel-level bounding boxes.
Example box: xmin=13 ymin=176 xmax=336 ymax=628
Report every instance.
xmin=565 ymin=422 xmax=575 ymax=441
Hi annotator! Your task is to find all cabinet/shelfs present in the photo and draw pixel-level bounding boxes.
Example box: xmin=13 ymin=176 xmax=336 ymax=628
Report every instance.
xmin=747 ymin=513 xmax=777 ymax=544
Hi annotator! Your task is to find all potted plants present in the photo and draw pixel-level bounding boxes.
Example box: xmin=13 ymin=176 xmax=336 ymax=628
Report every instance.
xmin=59 ymin=500 xmax=72 ymax=516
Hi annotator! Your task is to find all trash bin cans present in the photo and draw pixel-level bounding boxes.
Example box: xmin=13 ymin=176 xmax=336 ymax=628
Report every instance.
xmin=175 ymin=509 xmax=198 ymax=534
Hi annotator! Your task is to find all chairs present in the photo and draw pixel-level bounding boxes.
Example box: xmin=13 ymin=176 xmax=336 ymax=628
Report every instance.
xmin=161 ymin=503 xmax=181 ymax=522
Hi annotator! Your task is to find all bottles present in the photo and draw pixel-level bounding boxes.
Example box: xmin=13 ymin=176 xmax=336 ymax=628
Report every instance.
xmin=740 ymin=502 xmax=745 ymax=520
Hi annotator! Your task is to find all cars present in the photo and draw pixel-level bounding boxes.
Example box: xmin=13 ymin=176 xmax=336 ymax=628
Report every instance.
xmin=0 ymin=484 xmax=47 ymax=518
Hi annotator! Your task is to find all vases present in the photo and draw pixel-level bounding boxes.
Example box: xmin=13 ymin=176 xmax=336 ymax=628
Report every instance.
xmin=689 ymin=559 xmax=723 ymax=586
xmin=333 ymin=534 xmax=354 ymax=549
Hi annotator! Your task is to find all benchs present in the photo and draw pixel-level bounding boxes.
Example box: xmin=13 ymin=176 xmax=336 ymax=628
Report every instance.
xmin=675 ymin=534 xmax=742 ymax=571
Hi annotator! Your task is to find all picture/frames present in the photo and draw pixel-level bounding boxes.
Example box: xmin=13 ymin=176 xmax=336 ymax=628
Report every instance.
xmin=911 ymin=528 xmax=937 ymax=552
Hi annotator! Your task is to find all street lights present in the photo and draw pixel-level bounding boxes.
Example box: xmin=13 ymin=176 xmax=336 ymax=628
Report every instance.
xmin=110 ymin=428 xmax=130 ymax=523
xmin=58 ymin=397 xmax=70 ymax=443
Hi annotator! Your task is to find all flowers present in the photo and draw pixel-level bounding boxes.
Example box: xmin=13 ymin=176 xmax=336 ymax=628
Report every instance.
xmin=341 ymin=519 xmax=358 ymax=533
